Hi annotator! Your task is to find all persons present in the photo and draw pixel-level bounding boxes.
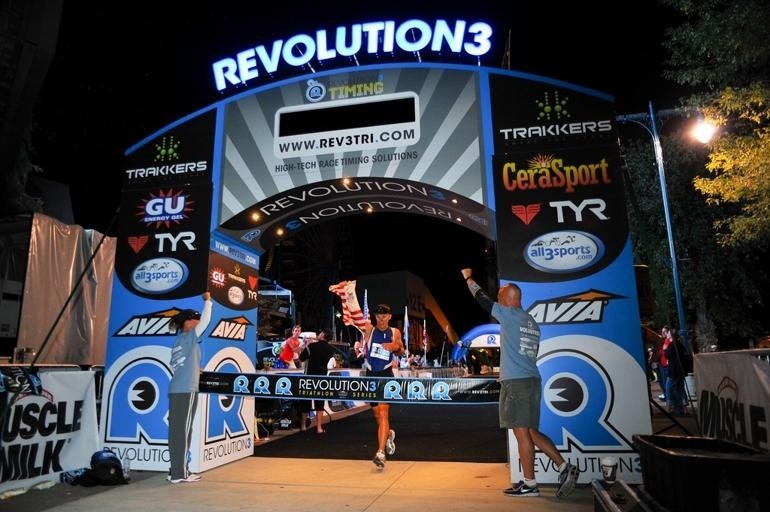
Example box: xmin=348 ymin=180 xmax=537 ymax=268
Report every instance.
xmin=274 ymin=324 xmax=442 ymax=372
xmin=168 ymin=292 xmax=212 ymax=485
xmin=345 ymin=281 xmax=407 ymax=468
xmin=460 ymin=268 xmax=580 ymax=500
xmin=646 ymin=326 xmax=691 ymax=417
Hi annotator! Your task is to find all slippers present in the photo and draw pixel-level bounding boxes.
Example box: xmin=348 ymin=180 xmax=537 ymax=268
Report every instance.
xmin=316 ymin=429 xmax=326 ymax=435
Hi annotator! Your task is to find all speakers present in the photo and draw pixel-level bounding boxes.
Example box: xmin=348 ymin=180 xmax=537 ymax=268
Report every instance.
xmin=634 ymin=264 xmax=655 ymax=321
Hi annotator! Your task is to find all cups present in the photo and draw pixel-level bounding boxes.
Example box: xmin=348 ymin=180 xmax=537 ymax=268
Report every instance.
xmin=601 ymin=456 xmax=618 ymax=483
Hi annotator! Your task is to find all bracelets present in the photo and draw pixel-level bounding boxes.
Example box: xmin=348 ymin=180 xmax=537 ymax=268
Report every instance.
xmin=395 ymin=346 xmax=402 ymax=354
xmin=204 ymin=297 xmax=212 ymax=301
xmin=464 ymin=275 xmax=472 ymax=281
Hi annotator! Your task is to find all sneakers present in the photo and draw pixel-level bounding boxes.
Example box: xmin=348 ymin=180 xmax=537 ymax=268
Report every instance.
xmin=386 ymin=430 xmax=396 ymax=455
xmin=503 ymin=480 xmax=539 ymax=497
xmin=373 ymin=453 xmax=385 ymax=473
xmin=166 ymin=475 xmax=201 ymax=484
xmin=554 ymin=464 xmax=579 ymax=499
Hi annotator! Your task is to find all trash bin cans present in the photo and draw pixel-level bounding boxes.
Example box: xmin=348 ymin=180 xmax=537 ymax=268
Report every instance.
xmin=683 ymin=373 xmax=698 ymax=401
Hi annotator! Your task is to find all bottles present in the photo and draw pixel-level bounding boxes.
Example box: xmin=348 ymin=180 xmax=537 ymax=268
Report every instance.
xmin=263 ymin=358 xmax=284 ymax=369
xmin=24 ymin=347 xmax=37 ymax=364
xmin=13 ymin=348 xmax=23 ymax=364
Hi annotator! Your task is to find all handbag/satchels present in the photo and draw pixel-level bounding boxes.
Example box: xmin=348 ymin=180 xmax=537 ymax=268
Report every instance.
xmin=60 ymin=468 xmax=89 ymax=486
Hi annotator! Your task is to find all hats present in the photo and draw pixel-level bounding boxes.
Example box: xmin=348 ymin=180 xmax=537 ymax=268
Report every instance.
xmin=369 ymin=306 xmax=392 ymax=315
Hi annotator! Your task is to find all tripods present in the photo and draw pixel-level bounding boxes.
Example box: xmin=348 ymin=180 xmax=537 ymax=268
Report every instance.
xmin=640 ymin=321 xmax=695 ymax=437
